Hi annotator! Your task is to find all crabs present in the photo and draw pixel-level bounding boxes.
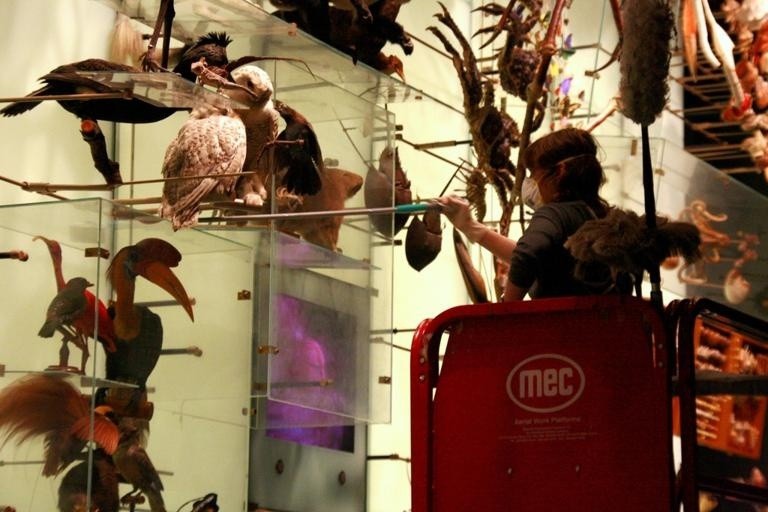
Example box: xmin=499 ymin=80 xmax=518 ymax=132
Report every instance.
xmin=470 ymin=0 xmax=548 ymax=134
xmin=425 ymin=0 xmax=517 ymax=212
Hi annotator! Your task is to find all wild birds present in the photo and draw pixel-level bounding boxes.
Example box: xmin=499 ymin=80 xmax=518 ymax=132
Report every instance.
xmin=271 ymin=1 xmax=415 ymax=84
xmin=0 ymin=236 xmax=194 ymax=512
xmin=0 ymin=31 xmax=325 ymax=232
xmin=379 ymin=145 xmax=408 ymax=188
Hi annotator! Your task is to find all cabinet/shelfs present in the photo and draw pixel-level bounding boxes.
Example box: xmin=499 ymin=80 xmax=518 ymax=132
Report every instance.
xmin=0 ymin=0 xmax=478 ymax=512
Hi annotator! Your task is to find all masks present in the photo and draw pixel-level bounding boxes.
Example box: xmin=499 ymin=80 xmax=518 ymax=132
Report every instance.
xmin=522 ymin=177 xmax=544 ymax=211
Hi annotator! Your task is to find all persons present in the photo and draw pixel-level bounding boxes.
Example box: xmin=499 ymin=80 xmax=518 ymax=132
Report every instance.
xmin=441 ymin=129 xmax=633 ymax=302
